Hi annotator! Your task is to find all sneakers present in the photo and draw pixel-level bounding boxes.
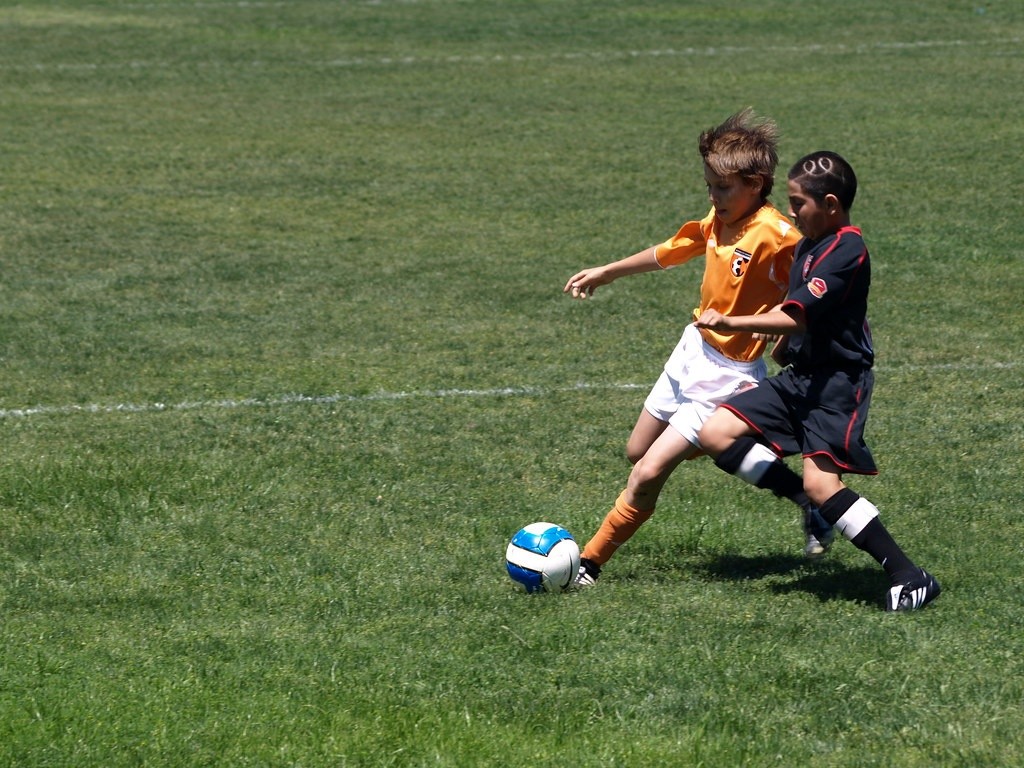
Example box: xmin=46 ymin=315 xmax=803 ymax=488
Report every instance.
xmin=801 ymin=508 xmax=834 ymax=559
xmin=887 ymin=568 xmax=941 ymax=612
xmin=568 ymin=557 xmax=602 ymax=589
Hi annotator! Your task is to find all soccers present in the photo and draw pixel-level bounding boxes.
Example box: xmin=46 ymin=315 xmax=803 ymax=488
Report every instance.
xmin=496 ymin=519 xmax=583 ymax=594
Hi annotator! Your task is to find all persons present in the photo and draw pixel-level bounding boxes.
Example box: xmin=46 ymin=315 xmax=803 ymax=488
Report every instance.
xmin=694 ymin=150 xmax=939 ymax=613
xmin=563 ymin=106 xmax=804 ymax=588
